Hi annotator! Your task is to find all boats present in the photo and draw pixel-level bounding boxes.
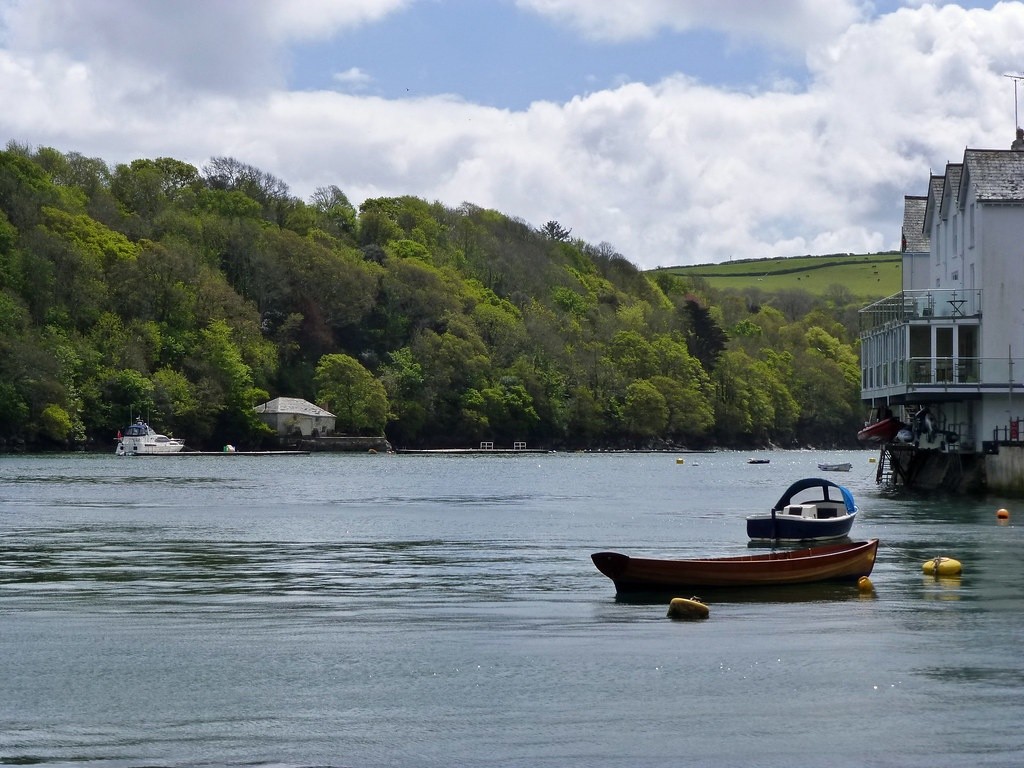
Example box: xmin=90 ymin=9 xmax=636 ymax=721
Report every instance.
xmin=590 ymin=538 xmax=882 ymax=594
xmin=818 ymin=462 xmax=853 ymax=471
xmin=745 ymin=477 xmax=859 ymax=542
xmin=114 ymin=416 xmax=186 ymax=457
xmin=747 ymin=458 xmax=770 ymax=464
